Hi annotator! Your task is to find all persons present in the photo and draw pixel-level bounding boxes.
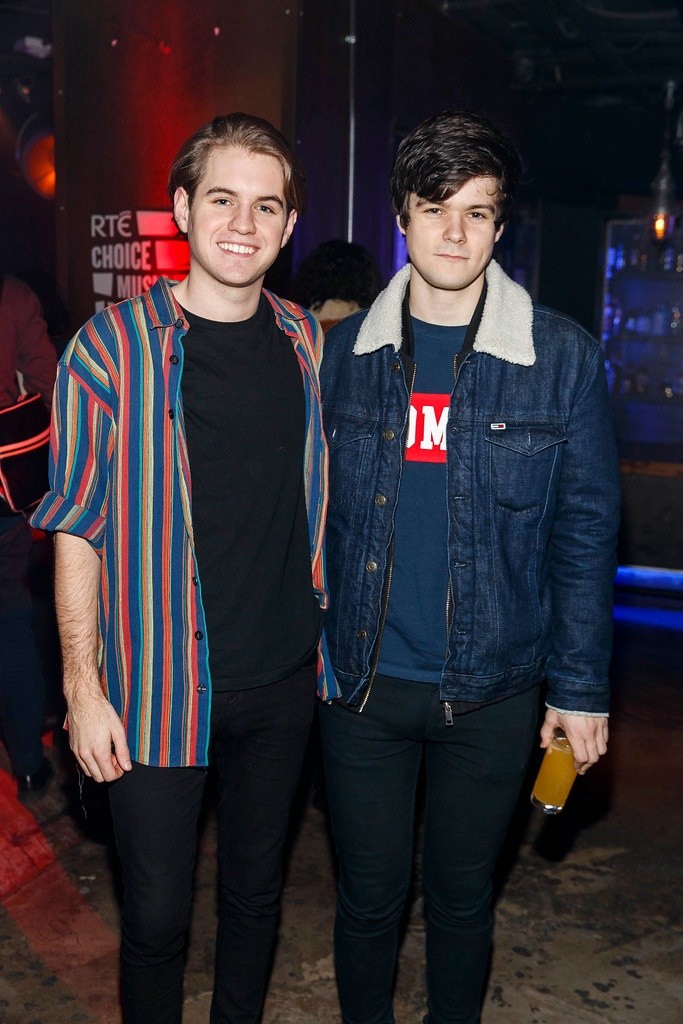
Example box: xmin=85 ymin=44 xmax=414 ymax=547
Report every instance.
xmin=269 ymin=239 xmax=387 ymax=338
xmin=27 ymin=112 xmax=342 ymax=1024
xmin=318 ymin=110 xmax=622 ymax=1024
xmin=0 ymin=276 xmax=58 ymax=801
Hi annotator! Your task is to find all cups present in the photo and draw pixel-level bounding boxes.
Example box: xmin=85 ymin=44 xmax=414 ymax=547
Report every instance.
xmin=530 ymin=731 xmax=588 ymax=814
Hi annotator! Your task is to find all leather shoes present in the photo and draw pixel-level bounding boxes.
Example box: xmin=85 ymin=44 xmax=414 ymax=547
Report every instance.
xmin=18 ymin=756 xmax=54 ymax=801
